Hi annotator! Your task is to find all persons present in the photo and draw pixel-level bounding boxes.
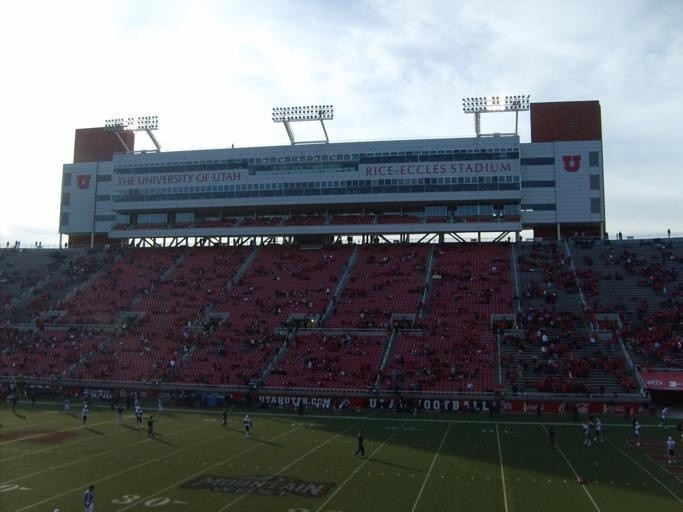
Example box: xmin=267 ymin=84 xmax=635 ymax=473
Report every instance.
xmin=0 ymin=228 xmax=682 ymax=464
xmin=82 ymin=484 xmax=96 ymax=512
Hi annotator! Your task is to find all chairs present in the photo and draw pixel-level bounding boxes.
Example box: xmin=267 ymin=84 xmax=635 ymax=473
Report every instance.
xmin=0 ymin=240 xmax=511 ymax=394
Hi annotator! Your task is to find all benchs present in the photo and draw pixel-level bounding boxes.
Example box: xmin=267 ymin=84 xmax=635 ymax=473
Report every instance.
xmin=513 ymin=237 xmax=682 ymax=396
